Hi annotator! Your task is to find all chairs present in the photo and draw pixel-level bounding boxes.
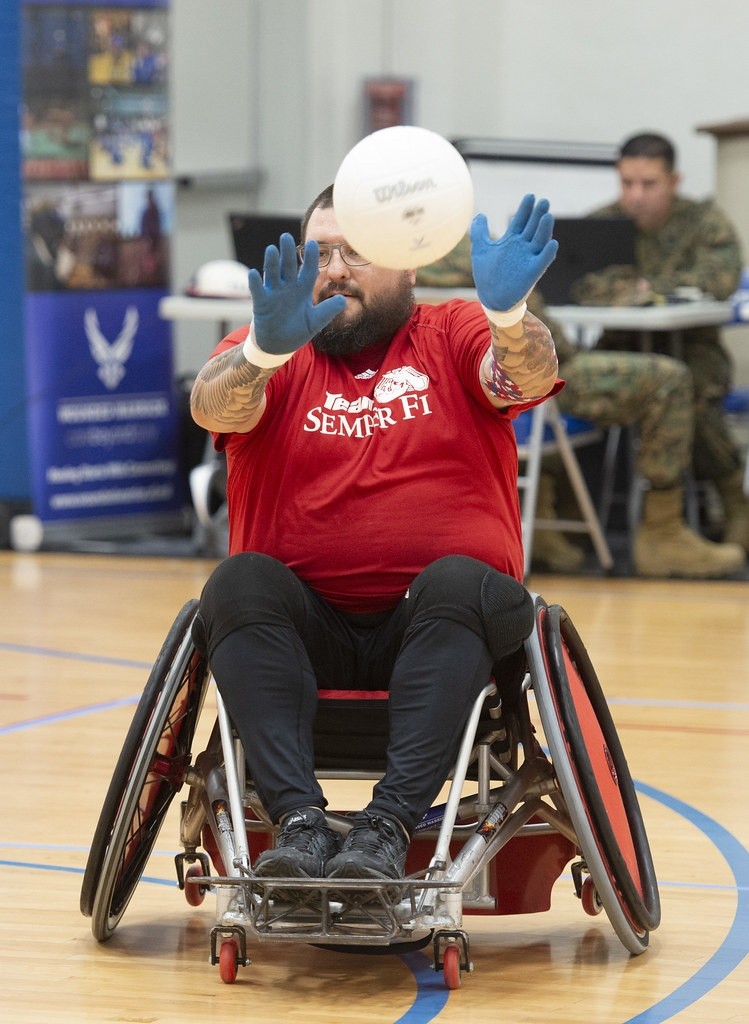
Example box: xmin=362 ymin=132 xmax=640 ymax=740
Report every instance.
xmin=628 ymin=390 xmax=749 ymax=537
xmin=511 ymin=351 xmax=616 ymax=580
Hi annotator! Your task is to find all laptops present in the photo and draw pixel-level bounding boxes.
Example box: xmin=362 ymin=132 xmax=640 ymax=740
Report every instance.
xmin=534 ymin=217 xmax=638 ymax=305
xmin=231 ymin=211 xmax=303 ymax=280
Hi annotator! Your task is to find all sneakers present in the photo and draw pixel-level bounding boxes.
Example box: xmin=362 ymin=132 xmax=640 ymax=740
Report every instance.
xmin=325 ymin=813 xmax=409 ymax=907
xmin=251 ymin=806 xmax=340 ymax=907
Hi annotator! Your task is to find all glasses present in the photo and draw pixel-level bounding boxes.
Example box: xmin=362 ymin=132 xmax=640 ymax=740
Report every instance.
xmin=298 ymin=241 xmax=369 ymax=267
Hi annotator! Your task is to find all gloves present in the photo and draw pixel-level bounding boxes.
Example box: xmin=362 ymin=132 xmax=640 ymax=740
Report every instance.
xmin=241 ymin=233 xmax=348 ymax=370
xmin=470 ymin=194 xmax=560 ymax=330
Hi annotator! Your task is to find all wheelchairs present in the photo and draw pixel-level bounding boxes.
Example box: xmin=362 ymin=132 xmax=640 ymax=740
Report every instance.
xmin=80 ymin=596 xmax=659 ymax=958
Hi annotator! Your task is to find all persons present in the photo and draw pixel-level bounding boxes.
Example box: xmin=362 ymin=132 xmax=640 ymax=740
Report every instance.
xmin=191 ymin=184 xmax=561 ymax=904
xmin=409 ymin=131 xmax=749 ymax=578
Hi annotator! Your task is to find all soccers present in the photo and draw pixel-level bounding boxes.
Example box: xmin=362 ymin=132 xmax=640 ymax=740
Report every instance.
xmin=332 ymin=125 xmax=474 ymax=269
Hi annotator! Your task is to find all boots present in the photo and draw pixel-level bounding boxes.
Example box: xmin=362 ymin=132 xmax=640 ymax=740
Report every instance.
xmin=711 ymin=465 xmax=748 ymax=555
xmin=631 ymin=487 xmax=746 ymax=579
xmin=519 ymin=474 xmax=590 ymax=573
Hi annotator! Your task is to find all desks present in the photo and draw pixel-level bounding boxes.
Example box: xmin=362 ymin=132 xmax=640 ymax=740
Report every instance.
xmin=156 ymin=288 xmax=736 ymax=576
